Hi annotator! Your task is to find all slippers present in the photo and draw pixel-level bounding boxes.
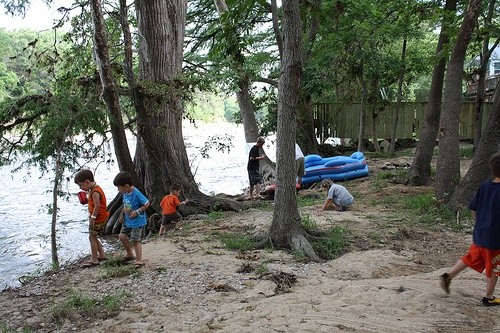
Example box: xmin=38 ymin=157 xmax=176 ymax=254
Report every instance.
xmin=82 ymin=262 xmax=100 ymax=267
xmin=134 ymin=264 xmax=145 ymax=270
xmin=98 ymin=258 xmax=107 ymax=261
xmin=121 ymin=257 xmax=133 ymax=262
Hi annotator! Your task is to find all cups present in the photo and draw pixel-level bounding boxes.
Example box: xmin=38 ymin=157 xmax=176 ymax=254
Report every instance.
xmin=78 ymin=192 xmax=88 ymax=205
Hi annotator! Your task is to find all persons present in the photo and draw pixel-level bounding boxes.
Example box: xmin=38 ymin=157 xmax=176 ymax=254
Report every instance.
xmin=160 ymin=183 xmax=188 ymax=236
xmin=319 ymin=179 xmax=354 ymax=212
xmin=114 ymin=171 xmax=150 ymax=266
xmin=247 ymin=137 xmax=265 ymax=199
xmin=440 ymin=153 xmax=500 ymax=307
xmin=295 ymin=143 xmax=305 ymax=185
xmin=74 ymin=170 xmax=107 ymax=267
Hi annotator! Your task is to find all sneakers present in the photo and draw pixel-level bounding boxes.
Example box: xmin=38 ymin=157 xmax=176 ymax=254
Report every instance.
xmin=439 ymin=273 xmax=451 ymax=294
xmin=482 ymin=295 xmax=500 ymax=306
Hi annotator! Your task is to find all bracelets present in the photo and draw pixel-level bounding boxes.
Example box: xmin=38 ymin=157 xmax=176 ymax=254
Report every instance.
xmin=91 ymin=214 xmax=96 ymax=219
xmin=136 ymin=209 xmax=142 ymax=214
xmin=183 ymin=201 xmax=185 ymax=205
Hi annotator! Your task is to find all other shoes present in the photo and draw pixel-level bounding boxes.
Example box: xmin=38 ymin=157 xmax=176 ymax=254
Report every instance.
xmin=336 ymin=206 xmax=350 ymax=211
xmin=255 ymin=194 xmax=265 ymax=200
